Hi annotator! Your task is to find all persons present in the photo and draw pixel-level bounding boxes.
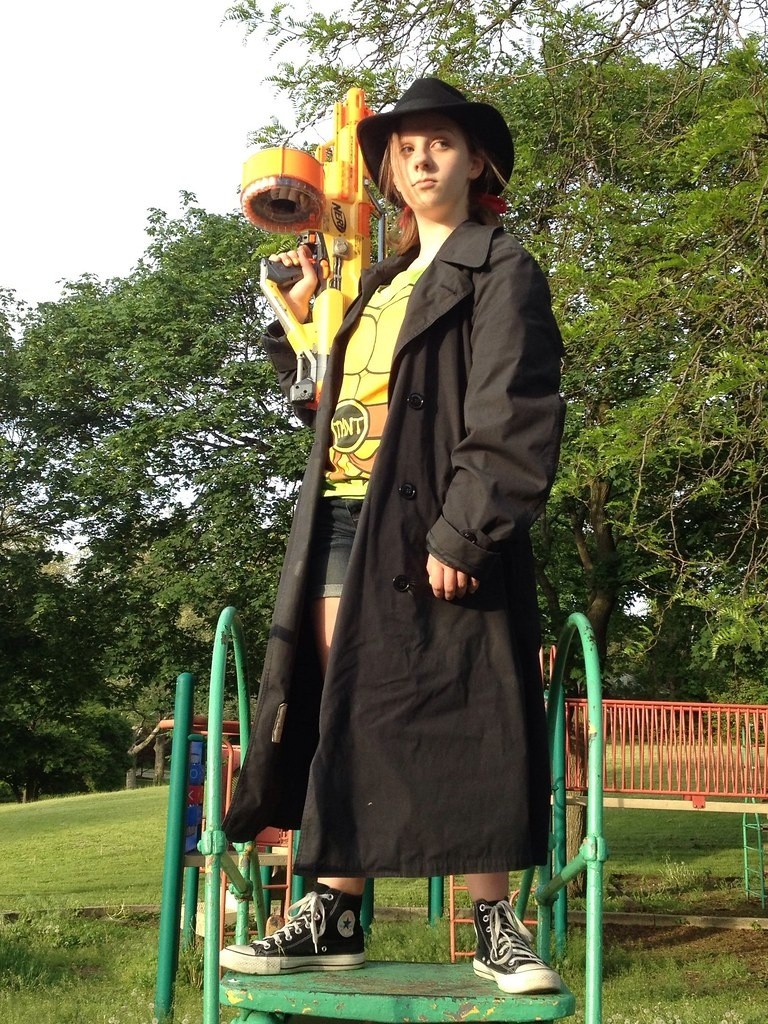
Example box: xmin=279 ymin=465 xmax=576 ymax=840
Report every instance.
xmin=220 ymin=81 xmax=561 ymax=993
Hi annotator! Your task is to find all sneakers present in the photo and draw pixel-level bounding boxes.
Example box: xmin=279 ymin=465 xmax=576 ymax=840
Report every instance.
xmin=471 ymin=898 xmax=563 ymax=994
xmin=220 ymin=880 xmax=366 ymax=973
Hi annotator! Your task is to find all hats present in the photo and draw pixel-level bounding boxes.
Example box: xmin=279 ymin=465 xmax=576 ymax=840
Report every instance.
xmin=357 ymin=78 xmax=515 ymax=209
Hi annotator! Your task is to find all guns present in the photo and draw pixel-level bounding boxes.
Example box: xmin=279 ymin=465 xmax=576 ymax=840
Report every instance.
xmin=237 ymin=86 xmax=378 ymax=410
